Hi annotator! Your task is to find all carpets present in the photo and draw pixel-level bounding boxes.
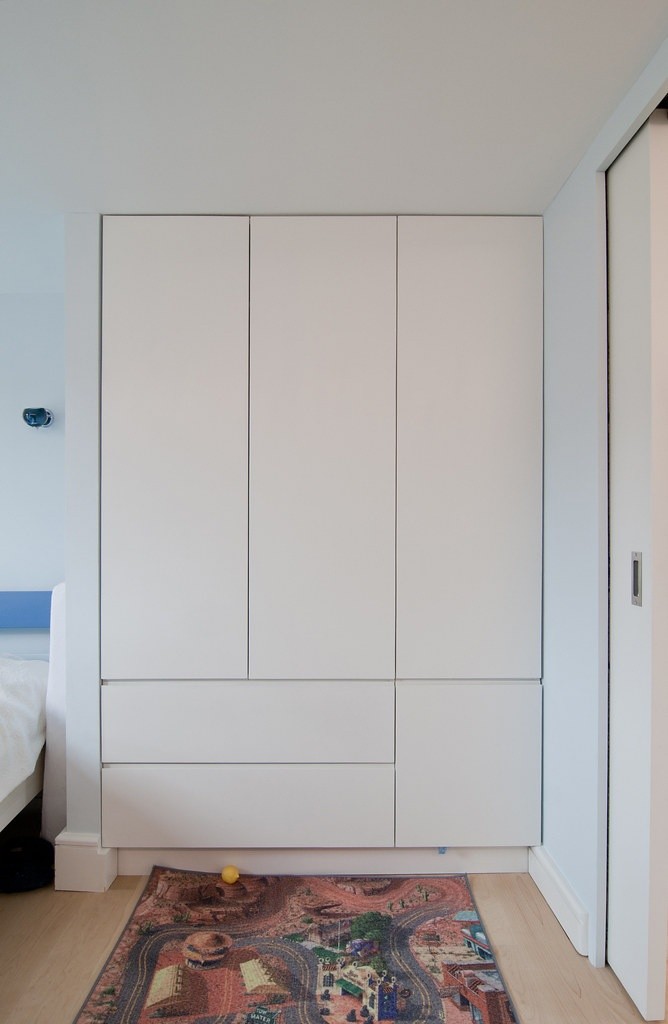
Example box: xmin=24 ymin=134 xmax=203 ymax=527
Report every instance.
xmin=72 ymin=863 xmax=521 ymax=1024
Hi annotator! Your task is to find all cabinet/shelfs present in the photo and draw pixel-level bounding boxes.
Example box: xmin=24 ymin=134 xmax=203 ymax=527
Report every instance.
xmin=50 ymin=186 xmax=550 ymax=896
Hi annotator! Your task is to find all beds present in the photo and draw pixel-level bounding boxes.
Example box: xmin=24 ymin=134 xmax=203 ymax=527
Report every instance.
xmin=0 ymin=581 xmax=53 ymax=832
